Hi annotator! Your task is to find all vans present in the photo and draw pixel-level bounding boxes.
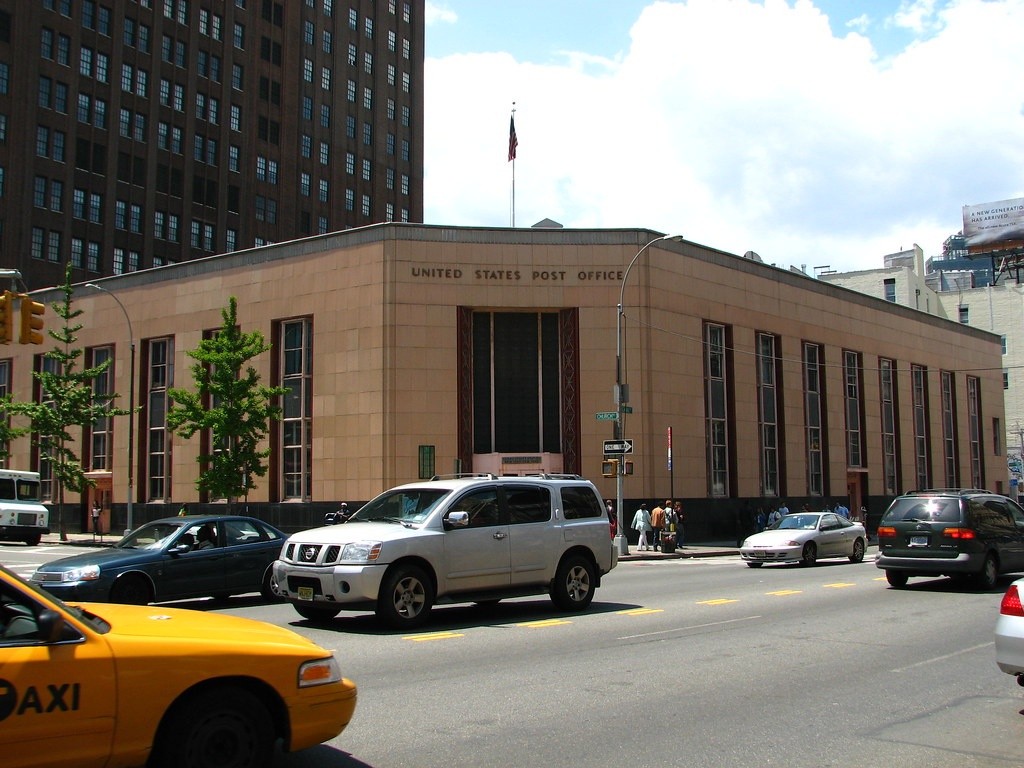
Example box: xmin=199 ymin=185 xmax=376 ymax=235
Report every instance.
xmin=0 ymin=469 xmax=50 ymax=546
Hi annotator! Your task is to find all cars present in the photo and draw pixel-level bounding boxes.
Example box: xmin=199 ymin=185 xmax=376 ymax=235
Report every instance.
xmin=0 ymin=565 xmax=358 ymax=768
xmin=28 ymin=515 xmax=294 ymax=605
xmin=994 ymin=577 xmax=1024 ymax=686
xmin=739 ymin=512 xmax=869 ymax=568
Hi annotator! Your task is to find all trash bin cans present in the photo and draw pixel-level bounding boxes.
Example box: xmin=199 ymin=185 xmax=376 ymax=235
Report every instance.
xmin=661 ymin=532 xmax=675 ymax=553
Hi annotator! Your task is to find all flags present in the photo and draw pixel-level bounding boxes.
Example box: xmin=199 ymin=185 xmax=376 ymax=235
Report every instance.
xmin=508 ymin=115 xmax=519 ymax=161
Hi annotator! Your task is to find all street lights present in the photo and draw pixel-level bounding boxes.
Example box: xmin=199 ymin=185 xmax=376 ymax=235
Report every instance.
xmin=85 ymin=283 xmax=137 ymax=548
xmin=613 ymin=233 xmax=684 ymax=555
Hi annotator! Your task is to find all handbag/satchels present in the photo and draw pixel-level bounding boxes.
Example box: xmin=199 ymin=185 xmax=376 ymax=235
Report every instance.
xmin=774 ymin=512 xmax=780 ymax=521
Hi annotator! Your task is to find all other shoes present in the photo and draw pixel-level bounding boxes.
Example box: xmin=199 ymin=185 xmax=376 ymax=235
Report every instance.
xmin=639 ymin=545 xmax=684 ymax=552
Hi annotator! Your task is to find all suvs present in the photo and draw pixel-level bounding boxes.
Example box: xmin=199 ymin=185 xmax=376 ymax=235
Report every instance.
xmin=272 ymin=472 xmax=619 ymax=630
xmin=875 ymin=488 xmax=1024 ymax=592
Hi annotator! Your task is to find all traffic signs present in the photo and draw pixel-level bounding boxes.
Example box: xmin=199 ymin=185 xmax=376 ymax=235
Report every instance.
xmin=603 ymin=439 xmax=633 ymax=454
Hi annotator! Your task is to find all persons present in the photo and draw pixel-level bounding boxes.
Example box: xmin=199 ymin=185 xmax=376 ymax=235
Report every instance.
xmin=606 ymin=500 xmax=616 ymax=539
xmin=650 ymin=499 xmax=686 ymax=552
xmin=630 ymin=504 xmax=653 ymax=551
xmin=822 ymin=501 xmax=850 ymax=519
xmin=192 ymin=525 xmax=214 ymax=551
xmin=91 ymin=501 xmax=103 ymax=534
xmin=801 ymin=503 xmax=812 ymax=512
xmin=337 ymin=503 xmax=352 ymax=524
xmin=753 ymin=500 xmax=789 ymax=532
xmin=178 ymin=502 xmax=191 ymax=515
